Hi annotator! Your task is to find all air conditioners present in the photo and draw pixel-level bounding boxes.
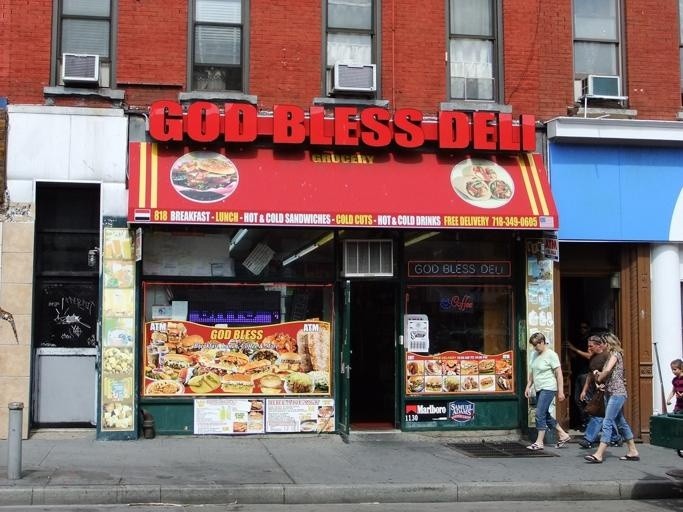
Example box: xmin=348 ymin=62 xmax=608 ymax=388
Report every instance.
xmin=61 ymin=53 xmax=102 ymax=87
xmin=581 ymin=74 xmax=620 ymax=96
xmin=343 ymin=236 xmax=395 ymax=278
xmin=329 ymin=63 xmax=376 ymax=92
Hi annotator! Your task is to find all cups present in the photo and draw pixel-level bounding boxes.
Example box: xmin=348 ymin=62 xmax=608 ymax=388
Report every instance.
xmin=147 ymin=341 xmax=170 ymax=368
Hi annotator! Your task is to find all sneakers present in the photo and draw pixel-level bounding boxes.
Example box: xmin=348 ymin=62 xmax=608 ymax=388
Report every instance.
xmin=610 ymin=440 xmax=623 ymax=447
xmin=555 ymin=436 xmax=571 ymax=448
xmin=526 ymin=443 xmax=544 ymax=450
xmin=577 ymin=439 xmax=591 ymax=448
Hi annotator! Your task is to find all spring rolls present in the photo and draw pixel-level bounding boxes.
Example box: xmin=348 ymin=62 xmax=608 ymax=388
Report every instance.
xmin=453 ymin=163 xmax=511 ymax=200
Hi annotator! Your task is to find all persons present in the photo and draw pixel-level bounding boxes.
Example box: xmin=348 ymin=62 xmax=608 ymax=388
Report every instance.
xmin=665 ymin=358 xmax=683 ymax=417
xmin=566 ymin=323 xmax=596 ymax=433
xmin=583 ymin=333 xmax=639 ymax=463
xmin=579 ymin=333 xmax=623 ymax=449
xmin=522 ymin=331 xmax=571 ymax=452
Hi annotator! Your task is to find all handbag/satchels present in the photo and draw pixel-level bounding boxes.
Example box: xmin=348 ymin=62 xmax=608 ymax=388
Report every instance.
xmin=529 ymin=384 xmax=536 ymax=397
xmin=584 ymin=395 xmax=605 ymax=417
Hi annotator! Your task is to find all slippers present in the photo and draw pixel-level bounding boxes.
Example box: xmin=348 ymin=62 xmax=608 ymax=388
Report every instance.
xmin=584 ymin=455 xmax=603 ymax=463
xmin=620 ymin=455 xmax=639 ymax=461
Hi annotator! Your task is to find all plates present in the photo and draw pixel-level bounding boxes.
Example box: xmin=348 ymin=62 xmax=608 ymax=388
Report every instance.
xmin=144 ymin=368 xmax=186 ymax=396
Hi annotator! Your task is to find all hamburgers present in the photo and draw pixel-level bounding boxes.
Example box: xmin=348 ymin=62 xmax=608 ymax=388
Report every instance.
xmin=481 ymin=377 xmax=494 ymax=388
xmin=151 ymin=332 xmax=328 ymax=394
xmin=427 ymin=377 xmax=442 ymax=391
